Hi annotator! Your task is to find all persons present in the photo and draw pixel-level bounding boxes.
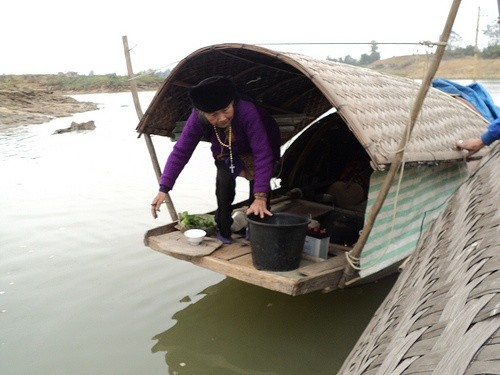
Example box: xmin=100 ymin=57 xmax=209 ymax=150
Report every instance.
xmin=458 ymin=118 xmax=500 ymax=162
xmin=151 ymin=76 xmax=280 ymax=244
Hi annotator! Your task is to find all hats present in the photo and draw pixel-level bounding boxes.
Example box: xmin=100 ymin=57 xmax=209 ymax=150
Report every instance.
xmin=189 ymin=75 xmax=235 ymax=114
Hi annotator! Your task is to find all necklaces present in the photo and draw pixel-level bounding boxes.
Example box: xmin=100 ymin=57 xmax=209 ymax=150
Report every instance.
xmin=214 ymin=125 xmax=235 ymax=174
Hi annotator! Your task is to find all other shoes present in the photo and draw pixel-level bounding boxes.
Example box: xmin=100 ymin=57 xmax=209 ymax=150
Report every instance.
xmin=217 ymin=232 xmax=232 ymax=244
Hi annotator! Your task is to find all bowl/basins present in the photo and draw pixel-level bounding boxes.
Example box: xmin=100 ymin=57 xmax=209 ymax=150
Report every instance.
xmin=184 ymin=229 xmax=206 ymax=245
xmin=178 ymin=214 xmax=217 ymax=237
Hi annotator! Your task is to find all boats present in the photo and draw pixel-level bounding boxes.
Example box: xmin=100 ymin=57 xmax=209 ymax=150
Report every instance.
xmin=114 ymin=41 xmax=500 ymax=298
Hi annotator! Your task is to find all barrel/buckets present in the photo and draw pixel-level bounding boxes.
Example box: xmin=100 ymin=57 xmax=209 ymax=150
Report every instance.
xmin=246 ymin=213 xmax=310 ymax=271
